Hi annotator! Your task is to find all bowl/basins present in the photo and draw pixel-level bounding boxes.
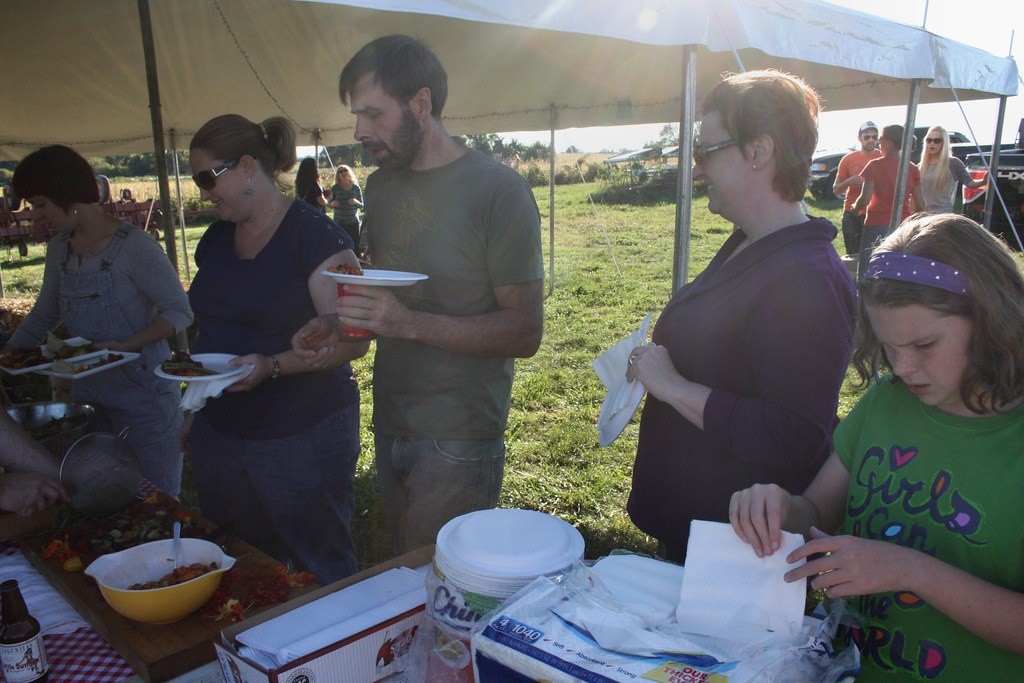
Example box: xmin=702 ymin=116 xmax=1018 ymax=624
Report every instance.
xmin=6 ymin=400 xmax=96 ymax=458
xmin=85 ymin=538 xmax=237 ymax=626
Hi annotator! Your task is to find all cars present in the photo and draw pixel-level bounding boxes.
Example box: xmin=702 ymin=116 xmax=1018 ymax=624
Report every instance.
xmin=808 ymin=126 xmax=973 ymax=202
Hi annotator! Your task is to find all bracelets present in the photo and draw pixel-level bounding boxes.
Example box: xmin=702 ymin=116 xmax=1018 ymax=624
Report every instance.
xmin=271 ymin=355 xmax=281 ymax=385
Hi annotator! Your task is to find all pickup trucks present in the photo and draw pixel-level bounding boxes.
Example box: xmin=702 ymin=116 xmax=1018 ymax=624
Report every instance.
xmin=948 ymin=118 xmax=1024 ymax=249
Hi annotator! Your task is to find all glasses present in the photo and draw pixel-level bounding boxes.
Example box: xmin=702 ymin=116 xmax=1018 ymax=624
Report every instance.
xmin=339 ymin=169 xmax=347 ymax=173
xmin=926 ymin=137 xmax=943 ymax=144
xmin=862 ymin=135 xmax=877 ymax=141
xmin=693 ymin=137 xmax=742 ymax=166
xmin=192 ymin=158 xmax=238 ymax=191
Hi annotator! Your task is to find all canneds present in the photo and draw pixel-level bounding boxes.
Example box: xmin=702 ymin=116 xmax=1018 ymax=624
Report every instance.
xmin=338 ymin=283 xmax=373 ymax=338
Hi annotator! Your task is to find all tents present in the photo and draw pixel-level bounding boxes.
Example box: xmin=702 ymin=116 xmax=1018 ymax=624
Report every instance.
xmin=1 ymin=0 xmax=1017 ymax=292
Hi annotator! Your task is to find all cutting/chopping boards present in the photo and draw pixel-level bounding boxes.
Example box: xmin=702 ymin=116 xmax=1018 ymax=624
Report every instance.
xmin=20 ymin=489 xmax=324 ymax=682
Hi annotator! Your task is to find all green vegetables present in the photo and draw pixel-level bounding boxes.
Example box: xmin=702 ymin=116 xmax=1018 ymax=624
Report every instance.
xmin=81 ymin=342 xmax=97 ymax=353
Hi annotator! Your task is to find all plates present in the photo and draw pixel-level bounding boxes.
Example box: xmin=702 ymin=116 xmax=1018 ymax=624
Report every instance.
xmin=0 ymin=336 xmax=142 ymax=381
xmin=599 ymin=341 xmax=656 ymax=446
xmin=434 ymin=509 xmax=586 ymax=607
xmin=154 ymin=353 xmax=250 ymax=381
xmin=321 ymin=268 xmax=430 ymax=287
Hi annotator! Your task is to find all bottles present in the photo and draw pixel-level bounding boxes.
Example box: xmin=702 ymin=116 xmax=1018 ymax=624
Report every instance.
xmin=0 ymin=580 xmax=50 ymax=683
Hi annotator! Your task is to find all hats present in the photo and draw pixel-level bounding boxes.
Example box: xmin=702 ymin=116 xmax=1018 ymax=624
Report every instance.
xmin=860 ymin=121 xmax=877 ymax=131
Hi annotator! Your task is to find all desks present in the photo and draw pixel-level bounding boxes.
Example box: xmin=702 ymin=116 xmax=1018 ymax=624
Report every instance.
xmin=0 ymin=392 xmax=323 ymax=683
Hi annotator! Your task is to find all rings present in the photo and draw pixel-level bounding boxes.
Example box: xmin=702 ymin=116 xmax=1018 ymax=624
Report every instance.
xmin=628 ymin=355 xmax=638 ymax=366
xmin=638 ymin=350 xmax=644 ymax=355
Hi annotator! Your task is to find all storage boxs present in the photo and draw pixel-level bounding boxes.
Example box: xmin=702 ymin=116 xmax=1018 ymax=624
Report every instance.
xmin=209 ymin=543 xmax=440 ymax=683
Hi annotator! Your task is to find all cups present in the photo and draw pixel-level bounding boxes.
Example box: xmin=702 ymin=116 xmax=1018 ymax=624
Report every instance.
xmin=337 ymin=283 xmax=379 ymax=340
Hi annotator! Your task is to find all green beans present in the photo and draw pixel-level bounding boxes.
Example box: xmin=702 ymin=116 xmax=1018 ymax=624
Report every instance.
xmin=161 ymin=361 xmax=221 ymax=374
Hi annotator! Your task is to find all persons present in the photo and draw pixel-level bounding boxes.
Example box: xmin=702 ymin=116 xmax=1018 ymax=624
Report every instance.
xmin=292 ymin=35 xmax=545 ymax=560
xmin=729 ymin=214 xmax=1024 ymax=683
xmin=833 ymin=121 xmax=989 ymax=274
xmin=185 ymin=113 xmax=372 ymax=579
xmin=0 ymin=142 xmax=195 ymax=516
xmin=295 ymin=157 xmax=364 ymax=258
xmin=625 ymin=71 xmax=857 ymax=565
